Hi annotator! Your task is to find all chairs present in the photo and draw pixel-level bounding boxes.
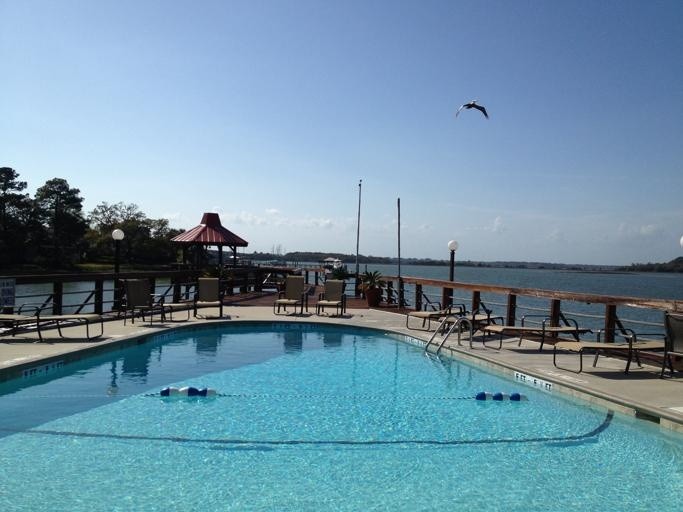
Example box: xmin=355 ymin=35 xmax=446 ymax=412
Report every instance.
xmin=273 ymin=276 xmax=308 ymax=315
xmin=314 ymin=279 xmax=347 ymax=317
xmin=552 ymin=328 xmax=673 ymax=375
xmin=437 ymin=306 xmax=504 ymax=336
xmin=0 ymin=276 xmax=223 ymax=343
xmin=482 ymin=315 xmax=593 ymax=352
xmin=405 ymin=301 xmax=469 ymax=332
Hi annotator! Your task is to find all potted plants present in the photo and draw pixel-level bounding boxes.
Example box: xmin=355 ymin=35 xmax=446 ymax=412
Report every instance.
xmin=358 ymin=271 xmax=386 ymax=307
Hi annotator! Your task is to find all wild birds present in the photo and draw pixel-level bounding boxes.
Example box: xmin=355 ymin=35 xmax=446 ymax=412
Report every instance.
xmin=455 ymin=99 xmax=490 ymax=121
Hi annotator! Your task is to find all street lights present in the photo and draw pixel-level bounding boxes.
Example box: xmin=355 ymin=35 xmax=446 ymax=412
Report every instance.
xmin=111 ymin=229 xmax=124 ymax=310
xmin=447 ymin=240 xmax=458 ymax=307
